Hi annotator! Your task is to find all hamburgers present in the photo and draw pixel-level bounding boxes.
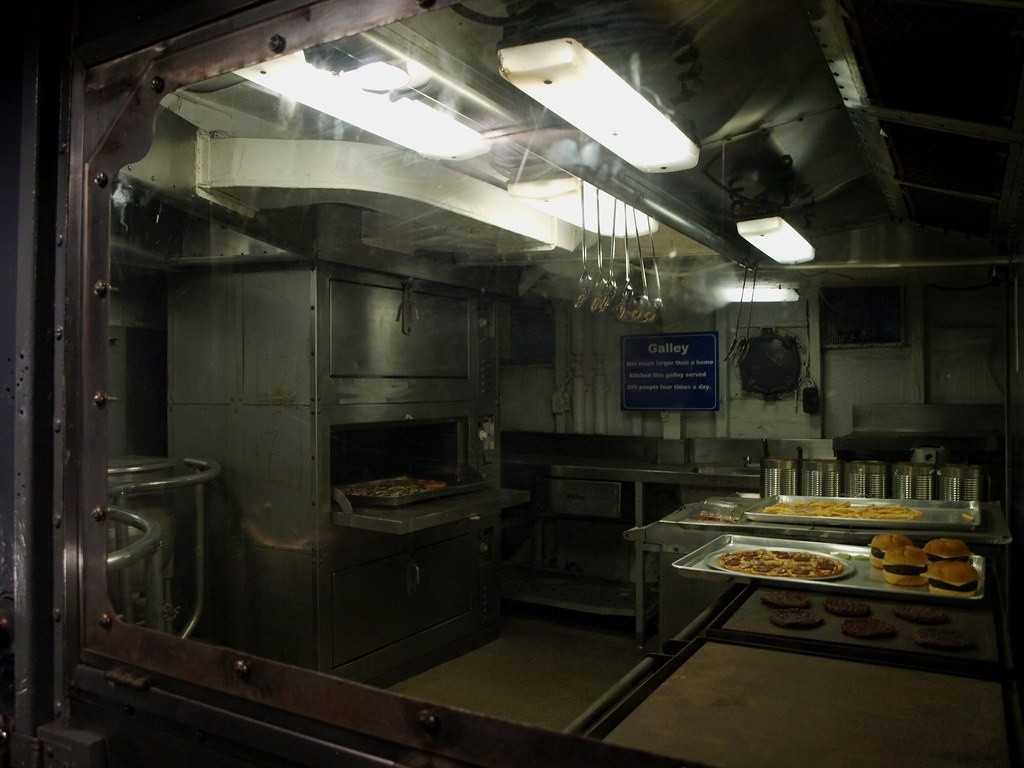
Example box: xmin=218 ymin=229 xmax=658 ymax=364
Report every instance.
xmin=867 ymin=533 xmax=979 ymax=597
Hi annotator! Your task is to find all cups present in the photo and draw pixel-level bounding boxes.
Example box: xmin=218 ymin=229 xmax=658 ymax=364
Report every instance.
xmin=702 ymin=498 xmax=745 ymax=523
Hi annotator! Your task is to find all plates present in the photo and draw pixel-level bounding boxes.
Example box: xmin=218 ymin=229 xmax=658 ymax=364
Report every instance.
xmin=706 ymin=547 xmax=855 ymax=581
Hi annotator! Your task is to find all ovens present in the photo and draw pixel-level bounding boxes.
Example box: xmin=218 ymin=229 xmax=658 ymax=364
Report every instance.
xmin=169 ymin=255 xmax=529 ymax=690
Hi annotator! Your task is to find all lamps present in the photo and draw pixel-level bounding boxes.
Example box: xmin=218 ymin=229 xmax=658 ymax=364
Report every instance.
xmin=492 ymin=0 xmax=702 ymax=174
xmin=728 ymin=153 xmax=818 ymax=266
xmin=502 ymin=163 xmax=659 ymax=239
xmin=709 ymin=277 xmax=802 ymax=303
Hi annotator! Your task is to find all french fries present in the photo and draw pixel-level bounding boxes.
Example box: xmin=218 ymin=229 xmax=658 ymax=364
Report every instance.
xmin=762 ymin=499 xmax=924 ymax=521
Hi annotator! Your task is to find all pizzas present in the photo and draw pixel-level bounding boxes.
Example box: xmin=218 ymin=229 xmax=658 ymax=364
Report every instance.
xmin=718 ymin=549 xmax=843 ymax=578
xmin=346 ymin=477 xmax=445 ymax=498
